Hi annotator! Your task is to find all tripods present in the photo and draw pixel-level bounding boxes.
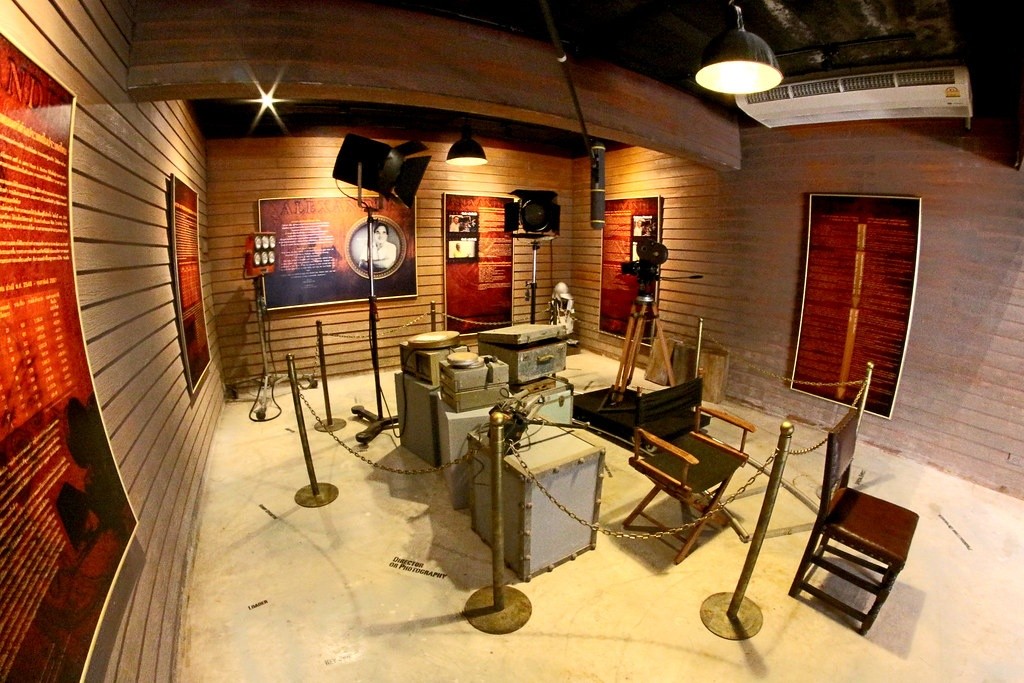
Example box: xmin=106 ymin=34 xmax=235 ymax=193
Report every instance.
xmin=611 ymin=261 xmax=703 ymax=407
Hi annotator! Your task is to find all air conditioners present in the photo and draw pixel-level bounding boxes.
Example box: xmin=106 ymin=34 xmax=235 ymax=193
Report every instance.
xmin=734 ymin=67 xmax=975 ymax=129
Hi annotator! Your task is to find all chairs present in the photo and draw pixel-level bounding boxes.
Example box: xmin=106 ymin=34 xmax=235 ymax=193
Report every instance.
xmin=629 ymin=368 xmax=757 ymax=565
xmin=788 ymin=409 xmax=921 ymax=635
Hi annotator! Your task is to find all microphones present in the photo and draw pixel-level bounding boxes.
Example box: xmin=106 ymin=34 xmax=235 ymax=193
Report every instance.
xmin=591 ymin=142 xmax=606 ymax=229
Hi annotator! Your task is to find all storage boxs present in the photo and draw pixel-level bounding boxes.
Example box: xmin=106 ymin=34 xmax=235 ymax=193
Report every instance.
xmin=477 ymin=323 xmax=568 ymax=385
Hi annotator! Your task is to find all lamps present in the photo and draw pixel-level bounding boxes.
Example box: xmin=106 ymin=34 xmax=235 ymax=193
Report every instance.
xmin=445 ymin=116 xmax=487 ymax=166
xmin=243 ymin=230 xmax=318 ymax=420
xmin=694 ymin=0 xmax=785 ymax=95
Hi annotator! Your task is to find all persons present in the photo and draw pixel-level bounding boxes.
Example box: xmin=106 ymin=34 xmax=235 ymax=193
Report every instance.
xmin=634 ymin=220 xmax=643 ymax=236
xmin=450 ymin=216 xmax=460 ymax=232
xmin=357 ymin=223 xmax=396 ymax=272
xmin=453 ymin=243 xmax=470 ymax=258
